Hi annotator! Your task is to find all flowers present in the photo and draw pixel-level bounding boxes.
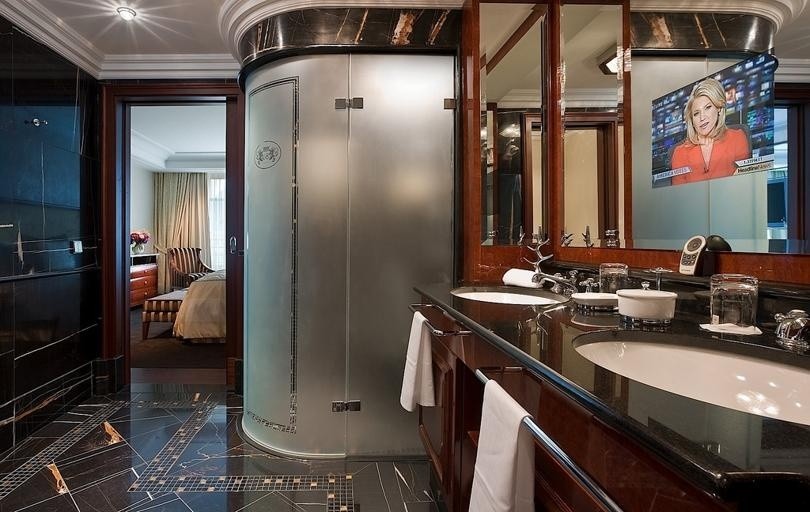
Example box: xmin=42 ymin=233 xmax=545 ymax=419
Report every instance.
xmin=130 ymin=232 xmax=147 ymax=243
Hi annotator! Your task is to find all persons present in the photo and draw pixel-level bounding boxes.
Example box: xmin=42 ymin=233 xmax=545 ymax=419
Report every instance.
xmin=668 ymin=77 xmax=754 ymax=183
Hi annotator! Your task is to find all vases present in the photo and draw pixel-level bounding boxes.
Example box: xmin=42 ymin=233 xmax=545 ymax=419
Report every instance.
xmin=134 ymin=244 xmax=142 ymax=254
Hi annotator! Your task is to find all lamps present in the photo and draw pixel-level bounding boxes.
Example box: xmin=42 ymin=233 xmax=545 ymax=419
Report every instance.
xmin=117 ymin=7 xmax=139 ymax=24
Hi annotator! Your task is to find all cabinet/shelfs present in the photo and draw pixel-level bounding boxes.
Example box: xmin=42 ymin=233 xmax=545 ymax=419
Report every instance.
xmin=417 ymin=292 xmax=718 ymax=511
xmin=129 ymin=253 xmax=158 ymax=307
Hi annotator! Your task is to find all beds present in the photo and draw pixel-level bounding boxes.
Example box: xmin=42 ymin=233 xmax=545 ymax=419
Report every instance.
xmin=188 ymin=270 xmax=226 ymax=344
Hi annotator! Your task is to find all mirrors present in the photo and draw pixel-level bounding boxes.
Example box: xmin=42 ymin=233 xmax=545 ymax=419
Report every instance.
xmin=629 ymin=2 xmax=809 ymax=255
xmin=478 ymin=12 xmax=549 ymax=248
xmin=556 ymin=3 xmax=631 ymax=250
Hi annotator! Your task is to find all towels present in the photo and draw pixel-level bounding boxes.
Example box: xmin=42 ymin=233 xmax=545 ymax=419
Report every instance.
xmin=400 ymin=307 xmax=539 ymax=512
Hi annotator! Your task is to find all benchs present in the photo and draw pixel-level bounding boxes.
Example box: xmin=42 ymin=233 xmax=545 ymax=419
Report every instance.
xmin=141 ymin=270 xmax=189 ymax=343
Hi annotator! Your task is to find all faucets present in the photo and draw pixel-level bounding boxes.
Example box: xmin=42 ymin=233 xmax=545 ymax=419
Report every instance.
xmin=774 ymin=308 xmax=810 ymax=355
xmin=532 ymin=271 xmax=580 ymax=304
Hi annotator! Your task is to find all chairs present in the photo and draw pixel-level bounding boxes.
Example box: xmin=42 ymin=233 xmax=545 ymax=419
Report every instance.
xmin=168 ymin=247 xmax=216 ymax=292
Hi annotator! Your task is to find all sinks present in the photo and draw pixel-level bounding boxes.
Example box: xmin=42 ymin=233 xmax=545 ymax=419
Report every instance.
xmin=569 ymin=325 xmax=810 ymax=428
xmin=449 ymin=283 xmax=571 ymax=308
